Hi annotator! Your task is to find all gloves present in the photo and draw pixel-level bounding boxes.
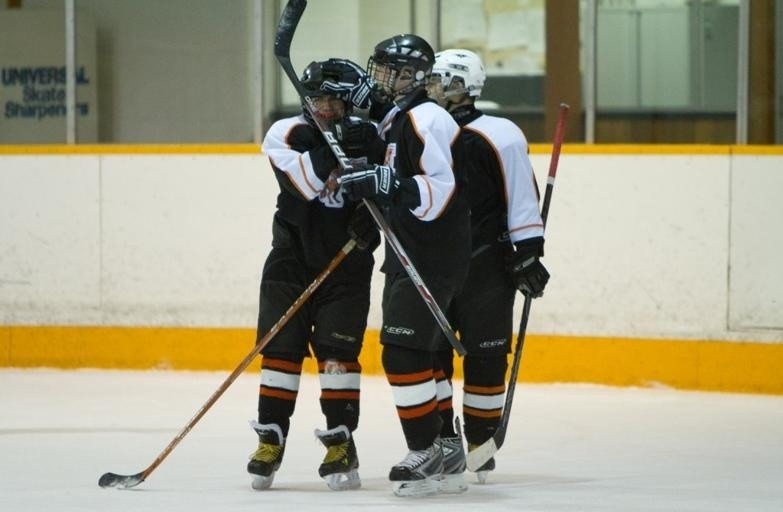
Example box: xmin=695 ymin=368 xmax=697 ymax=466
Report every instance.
xmin=334 ymin=120 xmax=400 ymax=250
xmin=513 ymin=256 xmax=550 ymax=299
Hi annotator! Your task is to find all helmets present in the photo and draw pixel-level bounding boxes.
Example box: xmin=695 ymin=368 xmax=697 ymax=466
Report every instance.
xmin=301 ymin=35 xmax=486 ymax=127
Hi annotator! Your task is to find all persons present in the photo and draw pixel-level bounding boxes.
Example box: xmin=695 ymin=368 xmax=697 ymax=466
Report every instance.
xmin=330 ymin=33 xmax=474 ymax=498
xmin=245 ymin=57 xmax=388 ymax=493
xmin=425 ymin=46 xmax=550 ymax=484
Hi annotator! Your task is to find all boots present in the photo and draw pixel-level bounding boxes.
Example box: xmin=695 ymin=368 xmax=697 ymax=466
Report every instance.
xmin=314 ymin=424 xmax=358 ymax=478
xmin=248 ymin=421 xmax=290 ymax=476
xmin=389 ymin=416 xmax=497 ymax=482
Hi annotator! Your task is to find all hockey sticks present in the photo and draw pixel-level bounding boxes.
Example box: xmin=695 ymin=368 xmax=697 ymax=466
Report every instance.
xmin=273 ymin=0 xmax=468 ymax=357
xmin=463 ymin=104 xmax=571 ymax=470
xmin=98 ymin=241 xmax=355 ymax=487
